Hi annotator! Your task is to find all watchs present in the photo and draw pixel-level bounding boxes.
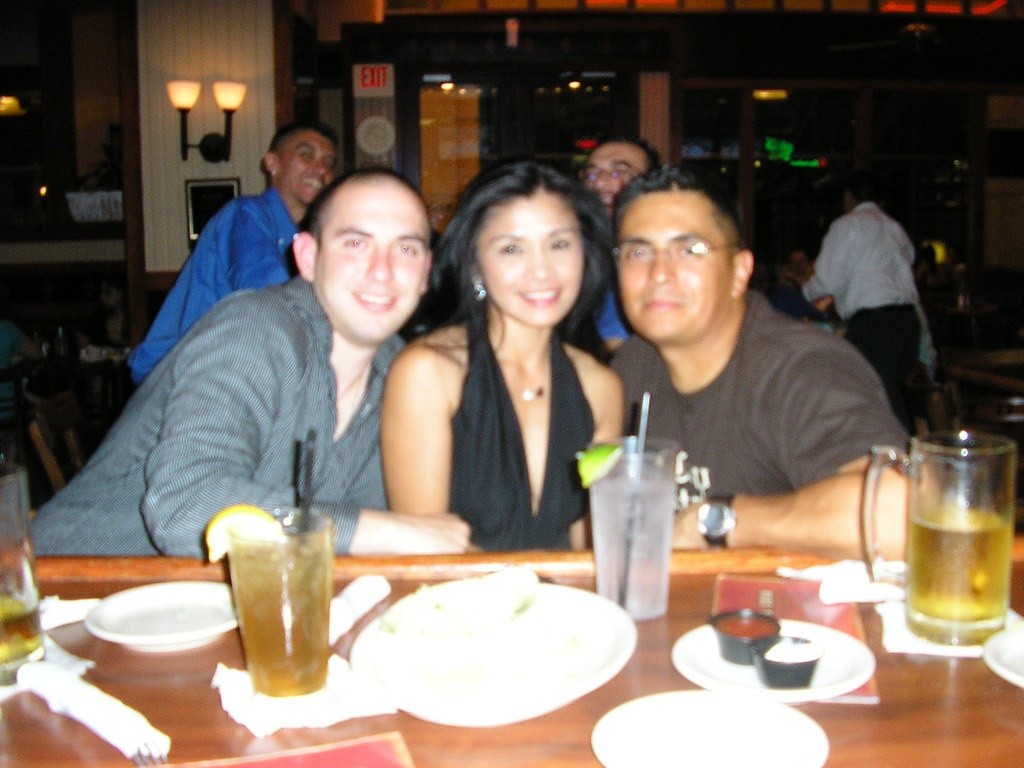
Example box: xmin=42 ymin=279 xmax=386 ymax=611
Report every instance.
xmin=698 ymin=488 xmax=737 ymax=549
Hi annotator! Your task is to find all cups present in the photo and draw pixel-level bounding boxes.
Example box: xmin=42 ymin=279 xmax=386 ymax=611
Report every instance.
xmin=860 ymin=433 xmax=1016 ymax=646
xmin=589 ymin=436 xmax=681 ymax=620
xmin=43 ymin=343 xmax=49 ymax=353
xmin=228 ymin=506 xmax=336 ymax=698
xmin=0 ymin=461 xmax=46 ymax=686
xmin=964 ymin=395 xmax=1024 ymax=533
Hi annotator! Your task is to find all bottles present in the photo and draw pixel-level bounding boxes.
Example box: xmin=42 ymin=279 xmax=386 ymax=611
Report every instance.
xmin=55 ymin=325 xmax=70 ymax=357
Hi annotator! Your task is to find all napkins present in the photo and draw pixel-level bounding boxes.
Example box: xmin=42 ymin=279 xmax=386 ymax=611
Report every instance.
xmin=327 ymin=576 xmax=389 ymax=648
xmin=776 ymin=558 xmax=906 ymax=606
xmin=17 ymin=662 xmax=171 ymax=760
xmin=212 ymin=650 xmax=399 ymax=738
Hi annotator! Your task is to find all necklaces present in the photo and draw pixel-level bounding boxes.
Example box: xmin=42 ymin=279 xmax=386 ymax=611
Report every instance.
xmin=522 ymin=385 xmax=545 ymax=400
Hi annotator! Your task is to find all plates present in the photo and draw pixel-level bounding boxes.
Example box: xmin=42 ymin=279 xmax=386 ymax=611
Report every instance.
xmin=84 ymin=581 xmax=239 ymax=652
xmin=671 ymin=620 xmax=876 ymax=704
xmin=349 ymin=578 xmax=637 ymax=727
xmin=983 ymin=623 xmax=1024 ymax=687
xmin=591 ymin=691 xmax=829 ymax=768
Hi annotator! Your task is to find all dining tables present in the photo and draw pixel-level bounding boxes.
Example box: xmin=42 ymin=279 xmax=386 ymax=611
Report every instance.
xmin=0 ymin=553 xmax=1024 ymax=768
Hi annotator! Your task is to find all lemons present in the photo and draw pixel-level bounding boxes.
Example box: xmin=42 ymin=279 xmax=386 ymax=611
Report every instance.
xmin=205 ymin=504 xmax=284 ymax=562
xmin=578 ymin=444 xmax=623 ymax=488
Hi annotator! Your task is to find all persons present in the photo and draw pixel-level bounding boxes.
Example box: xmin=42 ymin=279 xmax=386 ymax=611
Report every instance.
xmin=781 ymin=176 xmax=947 ymax=435
xmin=30 ymin=119 xmax=471 ymax=557
xmin=576 ymin=134 xmax=912 ymax=560
xmin=382 ymin=156 xmax=623 ymax=553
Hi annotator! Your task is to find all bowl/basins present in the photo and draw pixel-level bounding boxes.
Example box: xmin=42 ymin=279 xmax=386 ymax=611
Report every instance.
xmin=708 ymin=611 xmax=782 ymax=665
xmin=751 ymin=635 xmax=823 ymax=688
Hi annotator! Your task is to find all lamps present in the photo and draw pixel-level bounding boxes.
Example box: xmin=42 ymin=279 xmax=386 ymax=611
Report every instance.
xmin=165 ymin=80 xmax=247 ymax=164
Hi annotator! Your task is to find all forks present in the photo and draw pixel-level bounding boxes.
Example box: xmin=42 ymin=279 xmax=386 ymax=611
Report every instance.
xmin=133 ymin=744 xmax=168 ymax=768
